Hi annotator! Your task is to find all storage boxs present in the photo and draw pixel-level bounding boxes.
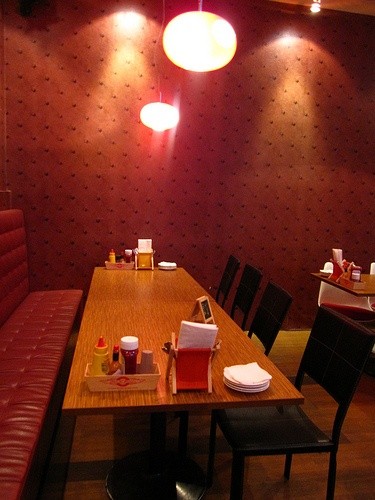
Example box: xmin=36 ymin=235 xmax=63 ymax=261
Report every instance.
xmin=85 ymin=361 xmax=164 ymax=392
xmin=340 ymin=277 xmax=366 ymax=289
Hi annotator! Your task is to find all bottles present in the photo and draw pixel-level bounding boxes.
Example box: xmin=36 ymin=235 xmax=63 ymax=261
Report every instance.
xmin=110 ymin=344 xmax=123 ymax=375
xmin=92 ymin=336 xmax=110 ymax=375
xmin=109 ymin=249 xmax=116 ymax=263
xmin=125 ymin=250 xmax=132 ymax=263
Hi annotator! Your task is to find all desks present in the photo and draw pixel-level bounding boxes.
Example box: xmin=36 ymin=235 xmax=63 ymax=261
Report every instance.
xmin=60 ymin=265 xmax=305 ymax=500
xmin=311 ymin=273 xmax=375 ymax=297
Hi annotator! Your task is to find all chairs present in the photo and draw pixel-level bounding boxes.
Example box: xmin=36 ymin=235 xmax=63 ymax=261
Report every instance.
xmin=231 ymin=264 xmax=262 ymax=331
xmin=370 ymin=263 xmax=375 ymax=275
xmin=318 ymin=282 xmax=371 ymax=312
xmin=208 ymin=305 xmax=375 ymax=500
xmin=176 ymin=281 xmax=292 ymax=456
xmin=216 ymin=255 xmax=241 ymax=308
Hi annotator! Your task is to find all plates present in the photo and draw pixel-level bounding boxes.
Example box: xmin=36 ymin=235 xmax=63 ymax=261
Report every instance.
xmin=158 ymin=262 xmax=177 ymax=270
xmin=320 ymin=270 xmax=332 ymax=276
xmin=223 ymin=365 xmax=269 ymax=392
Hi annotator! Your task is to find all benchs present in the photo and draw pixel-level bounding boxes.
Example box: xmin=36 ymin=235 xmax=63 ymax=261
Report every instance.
xmin=0 ymin=207 xmax=83 ymax=500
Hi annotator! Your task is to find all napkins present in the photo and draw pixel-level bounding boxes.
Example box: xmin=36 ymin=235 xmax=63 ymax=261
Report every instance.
xmin=227 ymin=362 xmax=272 ymax=386
xmin=333 ymin=248 xmax=342 ymax=267
